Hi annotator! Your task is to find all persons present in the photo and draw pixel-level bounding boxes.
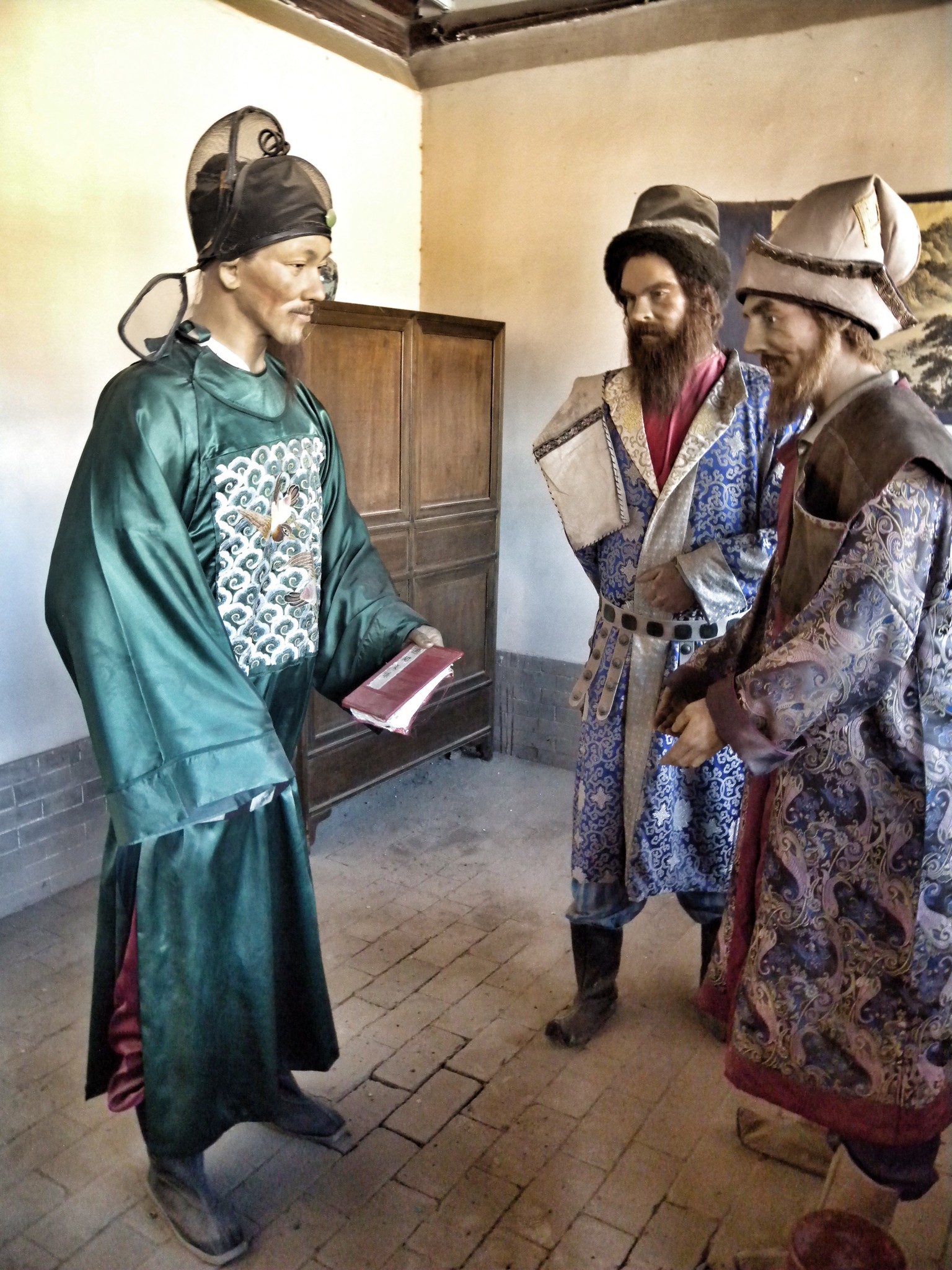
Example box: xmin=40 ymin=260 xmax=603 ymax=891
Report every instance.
xmin=653 ymin=172 xmax=952 ymax=1270
xmin=42 ymin=103 xmax=451 ymax=1270
xmin=533 ymin=187 xmax=811 ymax=1048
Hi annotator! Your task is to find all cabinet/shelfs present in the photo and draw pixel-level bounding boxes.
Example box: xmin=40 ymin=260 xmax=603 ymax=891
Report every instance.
xmin=263 ymin=304 xmax=508 ymax=847
